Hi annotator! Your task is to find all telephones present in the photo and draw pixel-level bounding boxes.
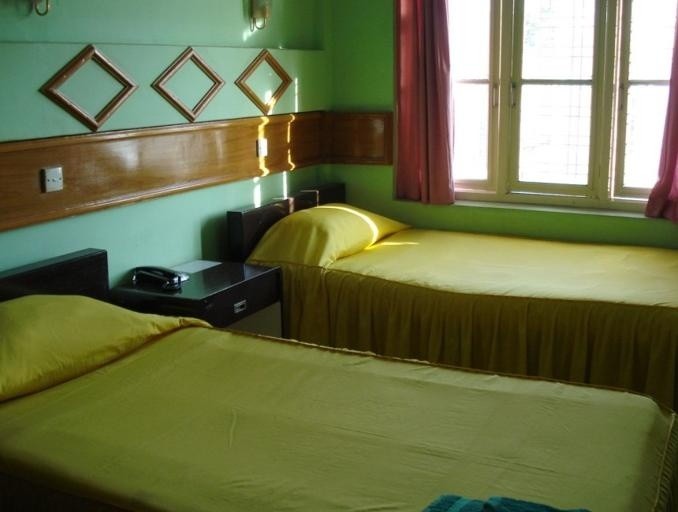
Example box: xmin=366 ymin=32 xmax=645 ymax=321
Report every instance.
xmin=135 ymin=265 xmax=183 ymax=291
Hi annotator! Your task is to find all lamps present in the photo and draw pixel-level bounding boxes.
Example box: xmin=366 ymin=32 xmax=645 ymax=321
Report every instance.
xmin=248 ymin=0 xmax=269 ymax=31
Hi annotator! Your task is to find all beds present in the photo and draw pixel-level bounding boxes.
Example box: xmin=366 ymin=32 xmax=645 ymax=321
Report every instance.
xmin=0 ymin=244 xmax=678 ymax=512
xmin=227 ymin=183 xmax=677 ymax=393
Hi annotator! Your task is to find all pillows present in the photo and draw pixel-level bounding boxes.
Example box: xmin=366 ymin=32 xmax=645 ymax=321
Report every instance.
xmin=250 ymin=201 xmax=408 ymax=267
xmin=0 ymin=292 xmax=175 ymax=400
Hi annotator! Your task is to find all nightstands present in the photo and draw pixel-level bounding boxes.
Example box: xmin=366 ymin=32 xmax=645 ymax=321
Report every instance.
xmin=114 ymin=259 xmax=285 ymax=336
